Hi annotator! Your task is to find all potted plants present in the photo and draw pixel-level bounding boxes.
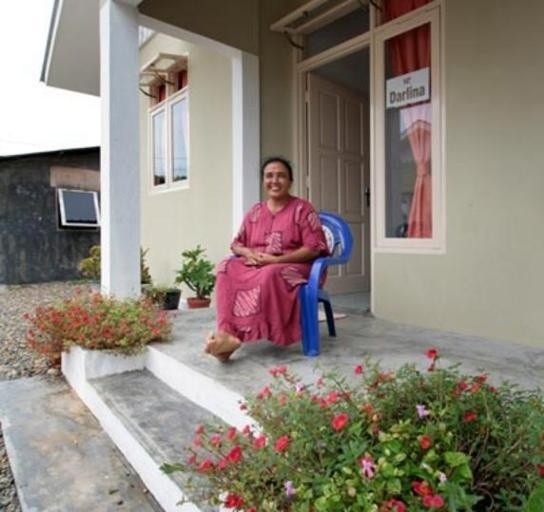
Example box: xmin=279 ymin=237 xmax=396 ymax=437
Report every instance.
xmin=175 ymin=246 xmax=217 ymax=309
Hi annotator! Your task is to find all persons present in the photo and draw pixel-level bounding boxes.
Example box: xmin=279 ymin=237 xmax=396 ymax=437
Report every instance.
xmin=204 ymin=157 xmax=330 ymax=364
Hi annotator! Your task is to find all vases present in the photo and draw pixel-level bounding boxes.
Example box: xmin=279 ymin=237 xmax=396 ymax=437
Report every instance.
xmin=156 ymin=288 xmax=180 ymax=310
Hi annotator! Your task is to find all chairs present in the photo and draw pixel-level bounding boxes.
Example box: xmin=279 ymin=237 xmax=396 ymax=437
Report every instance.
xmin=295 ymin=209 xmax=354 ymax=358
